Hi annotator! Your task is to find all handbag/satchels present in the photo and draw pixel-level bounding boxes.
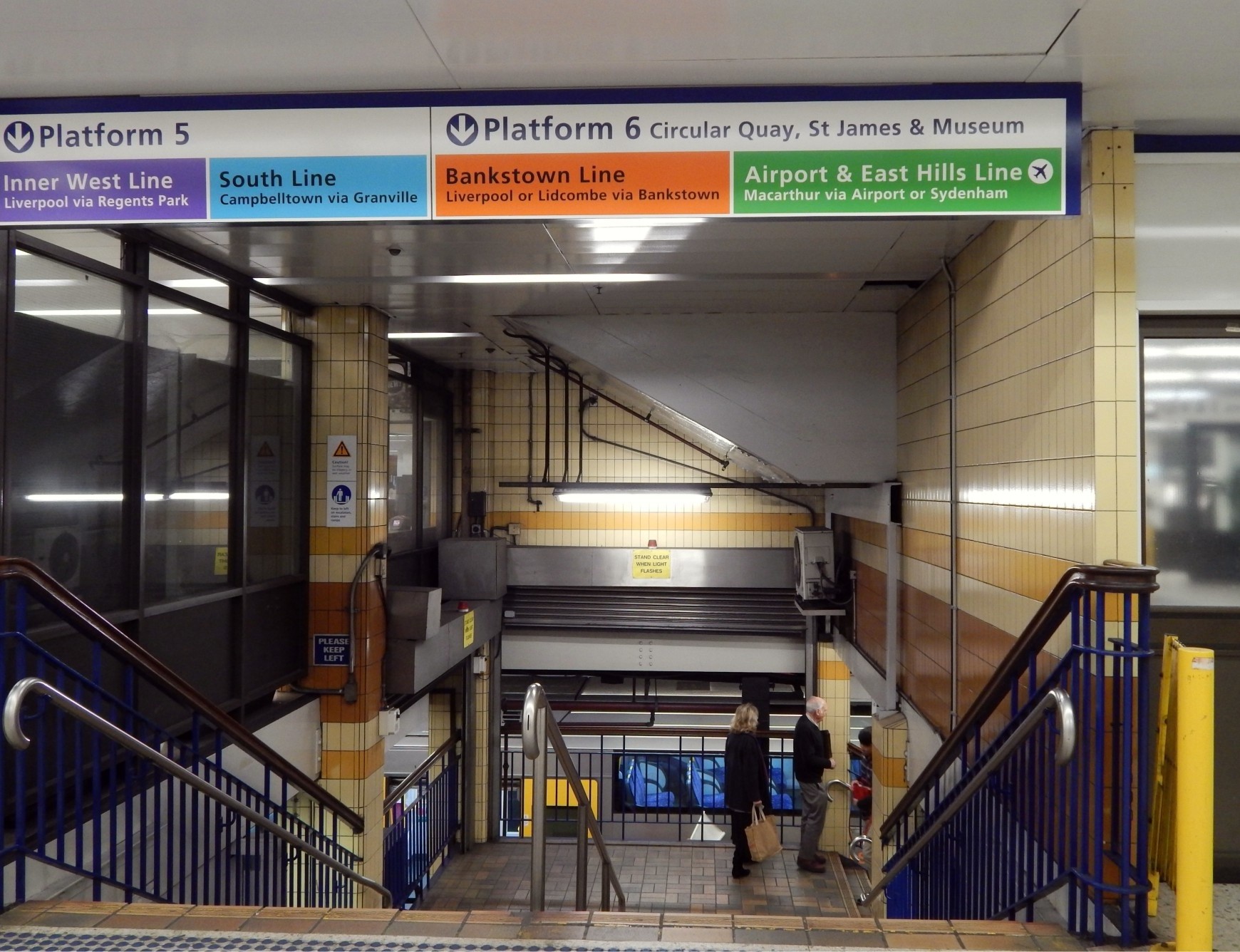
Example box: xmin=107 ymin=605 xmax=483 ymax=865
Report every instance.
xmin=744 ymin=804 xmax=783 ymax=862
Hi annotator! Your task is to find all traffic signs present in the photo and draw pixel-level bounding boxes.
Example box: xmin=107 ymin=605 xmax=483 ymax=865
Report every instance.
xmin=422 ymin=82 xmax=1085 ymax=219
xmin=0 ymin=88 xmax=432 ymax=229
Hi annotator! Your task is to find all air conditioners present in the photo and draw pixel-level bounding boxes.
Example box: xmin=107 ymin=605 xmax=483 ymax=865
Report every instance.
xmin=27 ymin=521 xmax=85 ymax=593
xmin=793 ymin=525 xmax=834 ymax=601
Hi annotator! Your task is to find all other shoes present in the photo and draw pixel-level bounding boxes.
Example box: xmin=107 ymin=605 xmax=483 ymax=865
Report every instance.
xmin=732 ymin=869 xmax=750 ymax=879
xmin=797 ymin=855 xmax=825 ymax=873
xmin=816 ymin=854 xmax=826 ymax=864
xmin=743 ymin=858 xmax=759 ymax=864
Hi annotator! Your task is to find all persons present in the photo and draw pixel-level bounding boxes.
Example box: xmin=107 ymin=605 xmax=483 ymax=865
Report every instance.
xmin=725 ymin=703 xmax=773 ymax=879
xmin=850 ymin=726 xmax=872 ymax=830
xmin=794 ymin=696 xmax=837 ymax=874
